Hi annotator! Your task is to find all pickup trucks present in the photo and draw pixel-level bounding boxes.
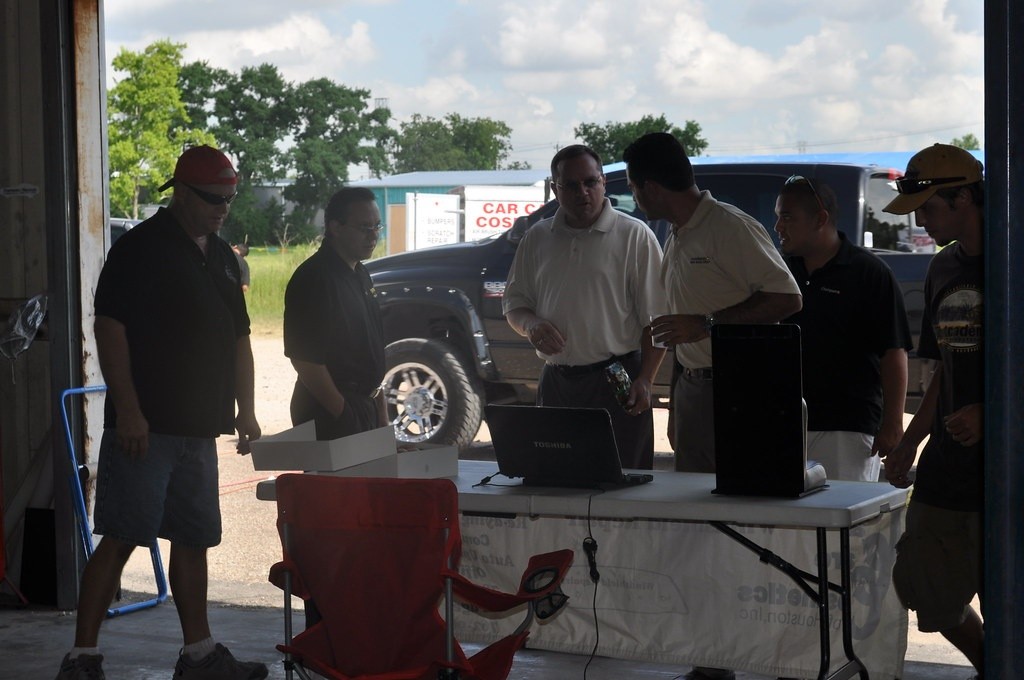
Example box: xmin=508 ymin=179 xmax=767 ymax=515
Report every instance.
xmin=362 ymin=163 xmax=940 ymax=454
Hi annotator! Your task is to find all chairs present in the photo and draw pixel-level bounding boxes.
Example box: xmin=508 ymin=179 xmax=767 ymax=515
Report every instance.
xmin=268 ymin=472 xmax=575 ymax=680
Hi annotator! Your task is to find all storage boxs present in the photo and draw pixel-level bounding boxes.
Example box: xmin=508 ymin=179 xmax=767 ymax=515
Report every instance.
xmin=249 ymin=420 xmax=397 ymax=470
xmin=302 ymin=441 xmax=460 ymax=479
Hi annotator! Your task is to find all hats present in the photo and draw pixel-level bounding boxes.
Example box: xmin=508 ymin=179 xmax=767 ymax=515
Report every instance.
xmin=158 ymin=144 xmax=239 ymax=192
xmin=882 ymin=143 xmax=983 ymax=215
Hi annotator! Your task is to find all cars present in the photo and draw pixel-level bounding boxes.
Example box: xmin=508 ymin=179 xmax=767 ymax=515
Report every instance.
xmin=109 ymin=216 xmax=143 ymax=245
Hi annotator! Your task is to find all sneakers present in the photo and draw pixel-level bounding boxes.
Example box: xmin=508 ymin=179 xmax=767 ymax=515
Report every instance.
xmin=172 ymin=642 xmax=269 ymax=680
xmin=55 ymin=652 xmax=106 ymax=680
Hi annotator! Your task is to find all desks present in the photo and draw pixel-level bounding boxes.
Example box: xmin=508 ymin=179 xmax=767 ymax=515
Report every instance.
xmin=256 ymin=458 xmax=913 ymax=680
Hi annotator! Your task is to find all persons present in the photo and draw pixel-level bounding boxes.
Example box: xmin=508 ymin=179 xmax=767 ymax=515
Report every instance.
xmin=55 ymin=144 xmax=270 ymax=680
xmin=774 ymin=172 xmax=915 ymax=481
xmin=284 ymin=187 xmax=389 ymax=631
xmin=881 ymin=144 xmax=988 ymax=680
xmin=501 ymin=145 xmax=669 ymax=470
xmin=622 ymin=132 xmax=804 ymax=680
xmin=230 ymin=243 xmax=250 ymax=292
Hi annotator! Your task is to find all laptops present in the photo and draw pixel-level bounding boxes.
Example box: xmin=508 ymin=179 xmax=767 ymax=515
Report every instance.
xmin=484 ymin=405 xmax=653 ymax=486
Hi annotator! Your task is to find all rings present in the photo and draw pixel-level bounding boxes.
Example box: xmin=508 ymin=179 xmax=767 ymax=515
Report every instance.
xmin=638 ymin=411 xmax=642 ymax=414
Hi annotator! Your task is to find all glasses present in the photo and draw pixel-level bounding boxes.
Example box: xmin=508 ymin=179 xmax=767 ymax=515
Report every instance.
xmin=181 ymin=182 xmax=238 ymax=204
xmin=785 ymin=174 xmax=831 ymax=224
xmin=557 ymin=175 xmax=603 ymax=192
xmin=343 ymin=223 xmax=384 ymax=233
xmin=894 ymin=176 xmax=966 ymax=194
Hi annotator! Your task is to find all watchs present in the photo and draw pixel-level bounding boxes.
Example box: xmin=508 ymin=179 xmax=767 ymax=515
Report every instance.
xmin=704 ymin=312 xmax=716 ymax=335
